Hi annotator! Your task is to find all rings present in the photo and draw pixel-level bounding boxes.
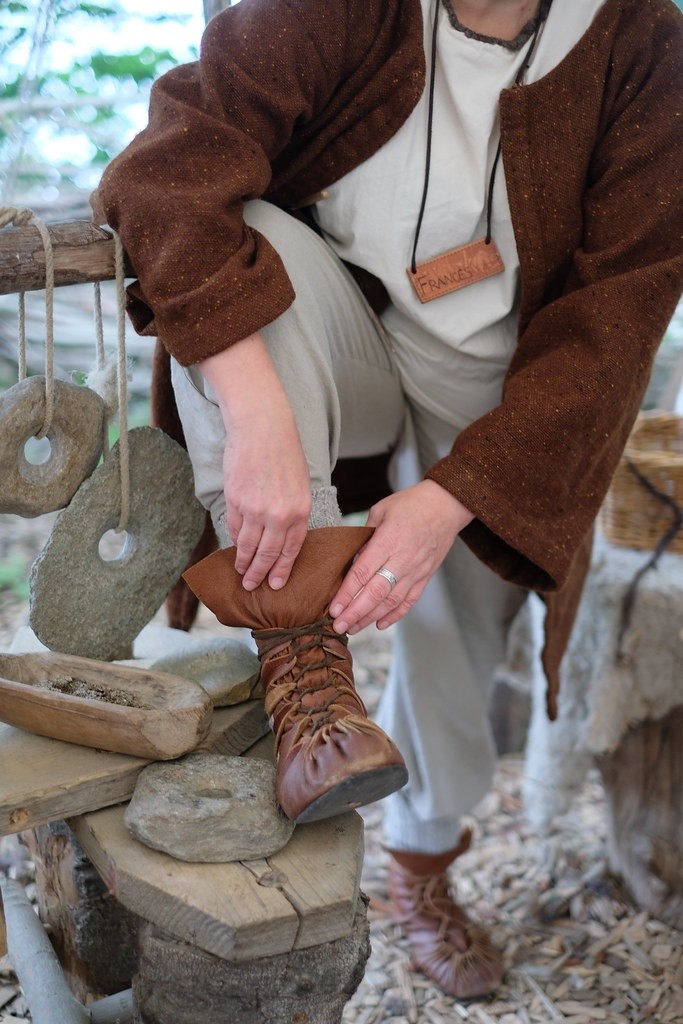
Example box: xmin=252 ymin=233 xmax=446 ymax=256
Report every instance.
xmin=376 ymin=567 xmax=397 ymax=590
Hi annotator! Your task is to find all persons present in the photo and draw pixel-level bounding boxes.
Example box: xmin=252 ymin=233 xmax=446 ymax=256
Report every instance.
xmin=88 ymin=1 xmax=683 ymax=1000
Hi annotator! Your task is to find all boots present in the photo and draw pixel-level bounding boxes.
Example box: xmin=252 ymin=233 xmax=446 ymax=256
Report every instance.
xmin=180 ymin=525 xmax=410 ymax=821
xmin=379 ymin=828 xmax=504 ymax=998
